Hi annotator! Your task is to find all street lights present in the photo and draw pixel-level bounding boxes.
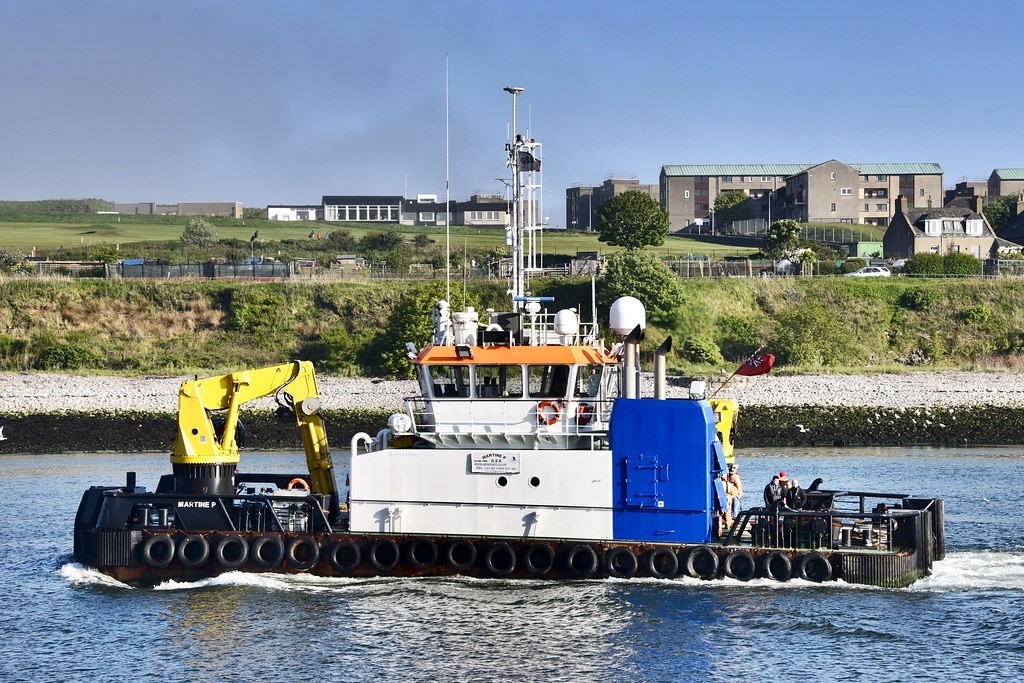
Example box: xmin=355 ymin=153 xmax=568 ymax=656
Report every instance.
xmin=546 ymin=191 xmax=552 ymax=232
xmin=768 ymin=189 xmax=775 ymax=231
xmin=586 ymin=190 xmax=594 ymax=231
xmin=564 ymin=196 xmax=576 ymax=230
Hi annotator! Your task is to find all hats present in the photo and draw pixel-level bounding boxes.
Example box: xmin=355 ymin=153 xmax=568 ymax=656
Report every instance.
xmin=780 ymin=471 xmax=786 ymax=477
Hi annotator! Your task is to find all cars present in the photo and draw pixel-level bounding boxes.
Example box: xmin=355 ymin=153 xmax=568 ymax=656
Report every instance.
xmin=844 ymin=266 xmax=891 ymax=278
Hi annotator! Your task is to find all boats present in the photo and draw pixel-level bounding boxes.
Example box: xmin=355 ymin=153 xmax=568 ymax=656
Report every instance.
xmin=71 ymin=59 xmax=947 ymax=592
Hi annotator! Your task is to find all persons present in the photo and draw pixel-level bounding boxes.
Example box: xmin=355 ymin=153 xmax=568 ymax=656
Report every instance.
xmin=309 ymin=228 xmax=329 ymax=240
xmin=764 ymin=471 xmax=807 ymax=518
xmin=714 ymin=464 xmax=743 ymax=535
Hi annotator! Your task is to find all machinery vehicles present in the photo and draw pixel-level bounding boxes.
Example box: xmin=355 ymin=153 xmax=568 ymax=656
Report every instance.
xmin=74 ymin=359 xmax=343 ymax=532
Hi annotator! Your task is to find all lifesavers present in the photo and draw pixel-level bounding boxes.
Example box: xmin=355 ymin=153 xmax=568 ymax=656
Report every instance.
xmin=143 ymin=534 xmax=177 ymax=569
xmin=686 ymin=546 xmax=720 ymax=581
xmin=285 ymin=535 xmax=319 ymax=570
xmin=647 ymin=547 xmax=679 ymax=579
xmin=536 ymin=401 xmax=560 ymax=425
xmin=217 ymin=535 xmax=249 ymax=568
xmin=370 ymin=538 xmax=399 ymax=570
xmin=723 ymin=549 xmax=756 ymax=581
xmin=567 ymin=545 xmax=597 ymax=578
xmin=330 ymin=540 xmax=362 ymax=572
xmin=446 ymin=539 xmax=477 ymax=570
xmin=607 ymin=547 xmax=638 ymax=578
xmin=799 ymin=553 xmax=832 ymax=583
xmin=486 ymin=541 xmax=516 ymax=574
xmin=409 ymin=538 xmax=439 ymax=569
xmin=762 ymin=551 xmax=791 ymax=581
xmin=287 ymin=478 xmax=308 ymax=490
xmin=178 ymin=534 xmax=210 ymax=568
xmin=251 ymin=535 xmax=284 ymax=567
xmin=524 ymin=543 xmax=556 ymax=575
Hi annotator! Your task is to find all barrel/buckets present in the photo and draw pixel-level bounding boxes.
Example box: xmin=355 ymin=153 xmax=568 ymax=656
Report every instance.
xmin=863 ymin=530 xmax=872 ymax=547
xmin=842 ymin=529 xmax=851 ymax=546
xmin=452 ymin=312 xmax=478 ymax=346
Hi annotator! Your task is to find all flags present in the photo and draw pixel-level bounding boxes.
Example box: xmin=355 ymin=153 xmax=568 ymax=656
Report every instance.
xmin=735 ymin=349 xmax=774 ymax=375
